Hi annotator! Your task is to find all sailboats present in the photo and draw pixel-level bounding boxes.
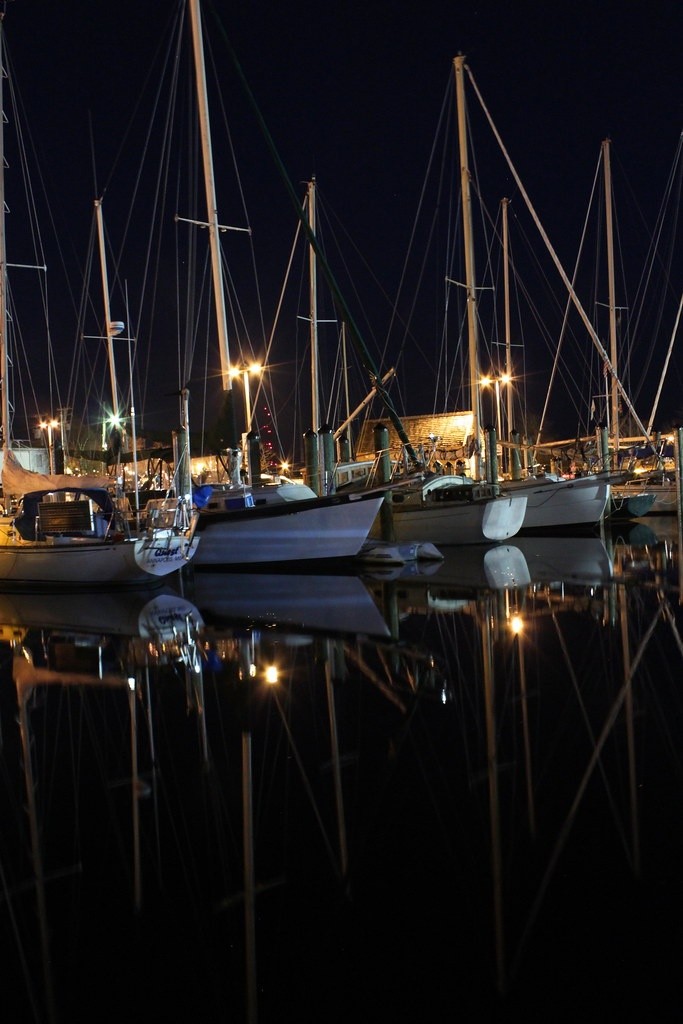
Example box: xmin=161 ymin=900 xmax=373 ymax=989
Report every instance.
xmin=1 ymin=0 xmax=682 ymax=580
xmin=0 ymin=542 xmax=683 ymax=1024
